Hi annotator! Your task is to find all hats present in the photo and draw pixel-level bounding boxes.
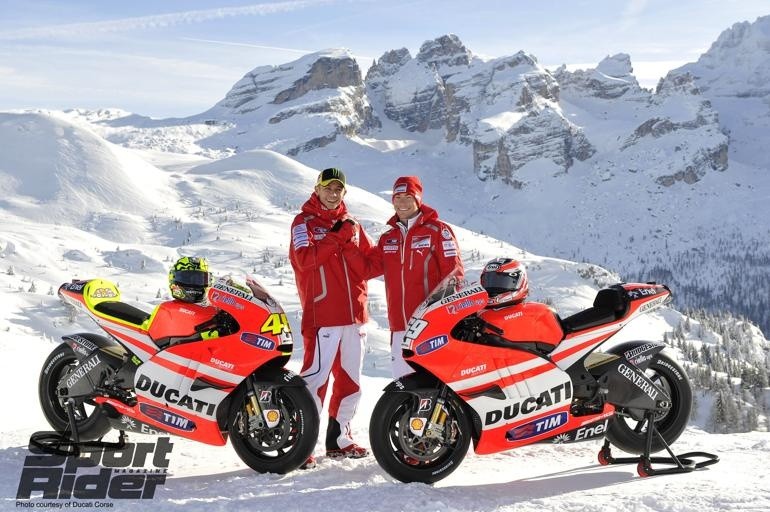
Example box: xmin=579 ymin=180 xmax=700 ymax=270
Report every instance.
xmin=392 ymin=176 xmax=423 ymax=208
xmin=317 ymin=167 xmax=345 ymax=188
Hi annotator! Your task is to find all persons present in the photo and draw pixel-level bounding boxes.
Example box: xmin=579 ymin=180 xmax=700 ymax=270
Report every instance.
xmin=335 ymin=175 xmax=465 ymax=465
xmin=288 ymin=168 xmax=374 ymax=471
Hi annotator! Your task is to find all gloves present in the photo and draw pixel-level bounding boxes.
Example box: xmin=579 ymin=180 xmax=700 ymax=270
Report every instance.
xmin=332 ymin=219 xmax=355 ymax=243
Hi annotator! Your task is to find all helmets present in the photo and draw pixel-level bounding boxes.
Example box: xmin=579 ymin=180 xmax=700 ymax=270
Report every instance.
xmin=480 ymin=258 xmax=528 ymax=309
xmin=169 ymin=257 xmax=214 ymax=302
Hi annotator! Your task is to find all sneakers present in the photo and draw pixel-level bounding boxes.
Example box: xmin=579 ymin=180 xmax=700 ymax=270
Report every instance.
xmin=299 ymin=455 xmax=316 ymax=470
xmin=326 ymin=445 xmax=367 ymax=461
xmin=405 ymin=457 xmax=430 ymax=465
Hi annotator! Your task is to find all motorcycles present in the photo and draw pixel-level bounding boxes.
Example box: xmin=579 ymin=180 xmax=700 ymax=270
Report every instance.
xmin=29 ymin=255 xmax=321 ymax=476
xmin=370 ymin=259 xmax=720 ymax=485
